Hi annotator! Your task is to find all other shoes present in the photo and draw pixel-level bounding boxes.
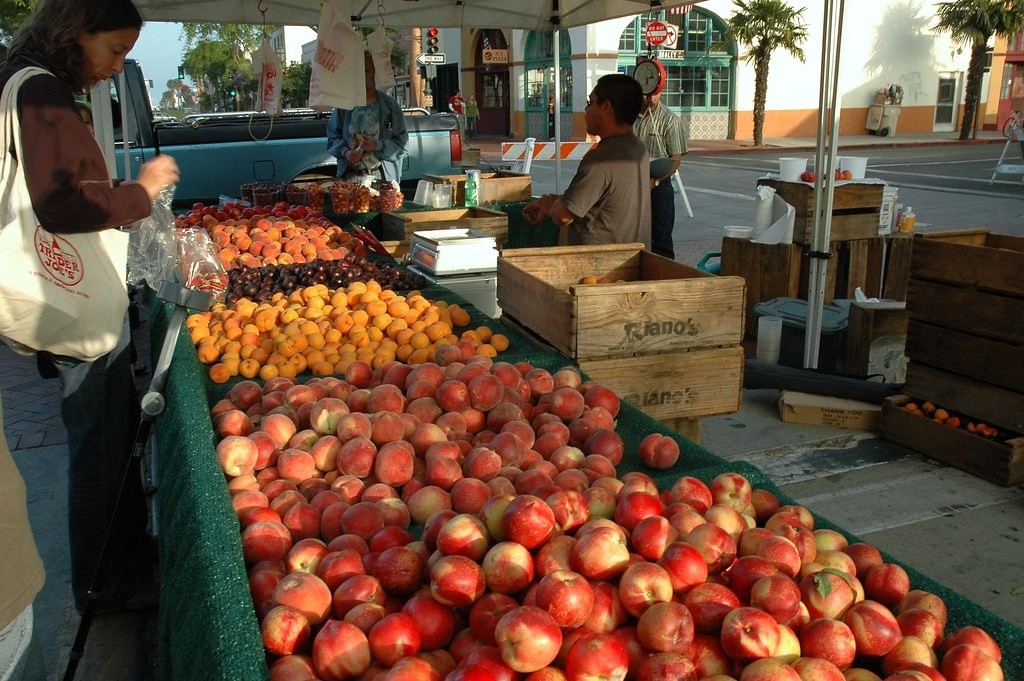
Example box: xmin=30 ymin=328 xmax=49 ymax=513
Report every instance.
xmin=78 ymin=577 xmax=160 ymax=614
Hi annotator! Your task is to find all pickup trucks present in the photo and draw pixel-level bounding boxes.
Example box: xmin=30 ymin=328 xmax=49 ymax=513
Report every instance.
xmin=66 ymin=59 xmax=466 ymax=214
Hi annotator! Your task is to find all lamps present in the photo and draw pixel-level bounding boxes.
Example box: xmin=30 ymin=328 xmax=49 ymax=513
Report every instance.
xmin=956 ymin=46 xmax=964 ymax=55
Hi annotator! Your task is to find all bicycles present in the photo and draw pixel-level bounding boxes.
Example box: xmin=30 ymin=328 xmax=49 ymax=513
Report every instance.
xmin=1002 ymin=110 xmax=1023 ymax=137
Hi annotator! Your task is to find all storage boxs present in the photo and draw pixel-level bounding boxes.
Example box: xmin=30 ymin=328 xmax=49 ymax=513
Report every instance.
xmin=778 ymin=387 xmax=880 ymax=429
xmin=497 ymin=243 xmax=749 ymax=358
xmin=876 ymin=91 xmax=902 ymax=105
xmin=578 ymin=342 xmax=744 ymax=421
xmin=904 ymin=318 xmax=1024 ymax=394
xmin=904 ymin=358 xmax=1024 ymax=435
xmin=903 ymin=279 xmax=1024 ymax=344
xmin=908 ymin=226 xmax=1024 ymax=298
xmin=417 ymin=168 xmax=533 ymax=205
xmin=878 ymin=393 xmax=1024 ymax=491
xmin=380 ymin=206 xmax=510 ymax=243
xmin=847 ymin=299 xmax=906 ymax=374
xmin=756 ymin=177 xmax=884 ymax=245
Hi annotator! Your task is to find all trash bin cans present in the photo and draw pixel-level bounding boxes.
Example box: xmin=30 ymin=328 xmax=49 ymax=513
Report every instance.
xmin=754 ymin=297 xmax=848 ymax=375
xmin=865 ymin=102 xmax=902 ymax=137
xmin=445 ymin=114 xmax=467 ymax=146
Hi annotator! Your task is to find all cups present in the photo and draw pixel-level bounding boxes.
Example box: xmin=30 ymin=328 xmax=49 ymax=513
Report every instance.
xmin=480 ymin=182 xmax=498 ymax=208
xmin=434 ymin=183 xmax=452 ymax=208
xmin=413 ymin=180 xmax=434 ymax=205
xmin=756 ymin=315 xmax=782 ymax=364
xmin=431 ymin=194 xmax=450 ymax=208
xmin=240 ymin=188 xmax=398 ymax=213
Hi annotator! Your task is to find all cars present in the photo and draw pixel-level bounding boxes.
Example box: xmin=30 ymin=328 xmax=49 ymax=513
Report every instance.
xmin=150 ymin=112 xmax=178 ymax=123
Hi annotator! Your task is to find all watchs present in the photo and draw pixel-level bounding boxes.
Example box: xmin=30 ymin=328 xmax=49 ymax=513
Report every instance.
xmin=654 ymin=180 xmax=659 ymax=187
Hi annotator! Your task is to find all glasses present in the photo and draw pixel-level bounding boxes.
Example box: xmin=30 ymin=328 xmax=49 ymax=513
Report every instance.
xmin=586 ymin=99 xmax=602 ymax=106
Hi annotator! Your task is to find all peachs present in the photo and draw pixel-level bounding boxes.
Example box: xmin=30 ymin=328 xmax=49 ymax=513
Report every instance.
xmin=581 ymin=276 xmax=627 ymax=284
xmin=207 ymin=342 xmax=681 ymax=562
xmin=185 ymin=280 xmax=509 ymax=383
xmin=901 ymin=401 xmax=997 ymax=436
xmin=172 ymin=201 xmax=368 ymax=269
xmin=231 ymin=473 xmax=1004 ymax=681
xmin=801 ymin=169 xmax=852 ymax=182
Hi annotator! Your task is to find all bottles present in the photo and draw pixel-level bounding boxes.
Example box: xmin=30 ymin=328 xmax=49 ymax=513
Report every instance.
xmin=355 ymin=141 xmax=365 ymax=155
xmin=465 ymin=173 xmax=478 ymax=207
xmin=900 ymin=207 xmax=915 ymax=235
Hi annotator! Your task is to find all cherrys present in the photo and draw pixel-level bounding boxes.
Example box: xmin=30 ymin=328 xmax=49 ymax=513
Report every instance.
xmin=240 ymin=181 xmax=403 ymax=214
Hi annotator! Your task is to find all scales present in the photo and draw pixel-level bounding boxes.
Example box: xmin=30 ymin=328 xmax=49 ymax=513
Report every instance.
xmin=410 ymin=228 xmax=499 ymax=275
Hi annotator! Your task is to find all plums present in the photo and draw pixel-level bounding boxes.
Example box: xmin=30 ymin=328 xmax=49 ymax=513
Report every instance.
xmin=225 ymin=255 xmax=424 ymax=302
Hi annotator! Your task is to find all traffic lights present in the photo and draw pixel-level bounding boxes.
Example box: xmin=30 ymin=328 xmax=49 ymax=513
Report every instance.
xmin=422 ymin=28 xmax=444 ymax=53
xmin=229 ymin=90 xmax=236 ymax=97
xmin=149 ymin=80 xmax=153 ymax=88
xmin=178 ymin=66 xmax=185 ymax=79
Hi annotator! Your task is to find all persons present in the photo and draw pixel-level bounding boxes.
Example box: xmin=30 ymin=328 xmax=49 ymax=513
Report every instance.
xmin=86 ymin=92 xmax=121 ymax=129
xmin=632 ymin=91 xmax=689 ymax=261
xmin=73 ymin=100 xmax=94 ymax=135
xmin=522 ymin=74 xmax=652 ymax=253
xmin=0 ymin=392 xmax=47 ymax=681
xmin=1 ymin=0 xmax=180 ymax=626
xmin=325 ymin=48 xmax=408 ymax=191
xmin=448 ymin=90 xmax=466 ymax=114
xmin=464 ymin=93 xmax=480 ymax=140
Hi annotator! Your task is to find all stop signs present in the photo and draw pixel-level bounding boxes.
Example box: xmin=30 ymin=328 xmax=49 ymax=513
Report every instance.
xmin=646 ymin=22 xmax=668 ymax=45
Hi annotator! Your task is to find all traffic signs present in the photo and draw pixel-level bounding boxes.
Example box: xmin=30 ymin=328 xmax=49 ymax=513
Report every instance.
xmin=415 ymin=53 xmax=447 ymax=65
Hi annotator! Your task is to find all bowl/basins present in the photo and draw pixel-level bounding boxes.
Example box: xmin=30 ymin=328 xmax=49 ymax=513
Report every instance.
xmin=813 ymin=154 xmax=840 ymax=175
xmin=840 ymin=157 xmax=867 ymax=181
xmin=778 ymin=158 xmax=808 ymax=181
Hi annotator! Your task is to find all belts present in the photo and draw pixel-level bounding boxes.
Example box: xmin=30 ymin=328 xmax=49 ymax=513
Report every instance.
xmin=349 ymin=167 xmax=380 ymax=176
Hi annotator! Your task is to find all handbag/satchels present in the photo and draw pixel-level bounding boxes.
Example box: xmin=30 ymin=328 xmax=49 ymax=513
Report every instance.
xmin=0 ymin=66 xmax=130 ymax=362
